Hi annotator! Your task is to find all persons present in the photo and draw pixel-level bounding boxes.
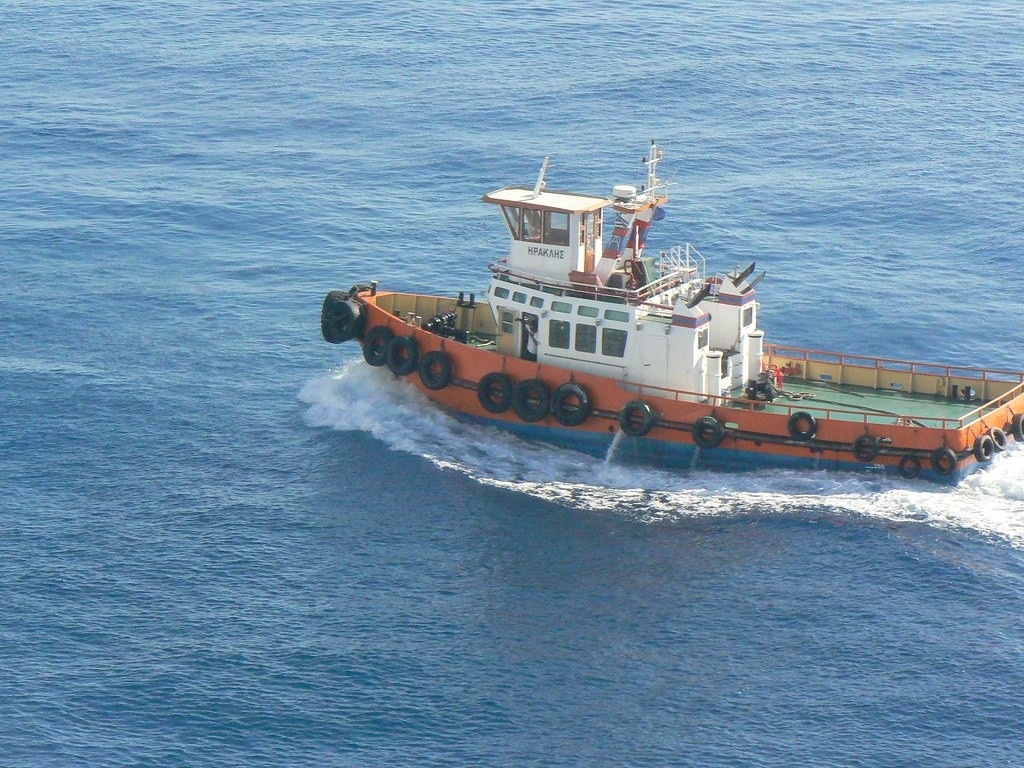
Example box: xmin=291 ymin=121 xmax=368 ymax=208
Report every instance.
xmin=515 ymin=317 xmax=539 ymax=361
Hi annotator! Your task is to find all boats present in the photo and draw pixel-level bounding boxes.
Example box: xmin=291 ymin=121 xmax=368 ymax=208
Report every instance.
xmin=320 ymin=139 xmax=1024 ymax=486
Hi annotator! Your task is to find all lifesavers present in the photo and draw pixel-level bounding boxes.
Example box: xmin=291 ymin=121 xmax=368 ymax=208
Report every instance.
xmin=418 ymin=350 xmax=456 ymax=391
xmin=362 ymin=326 xmax=395 ymax=366
xmin=988 ymin=427 xmax=1008 ymax=452
xmin=787 ymin=411 xmax=817 ymax=441
xmin=386 ymin=333 xmax=418 ymax=376
xmin=478 ymin=371 xmax=512 ymax=414
xmin=329 ymin=299 xmax=361 ymax=336
xmin=692 ymin=416 xmax=725 ymax=449
xmin=973 ymin=434 xmax=994 ymax=463
xmin=511 ymin=380 xmax=551 ymax=422
xmin=551 ymin=383 xmax=591 ymax=425
xmin=1011 ymin=413 xmax=1024 ymax=443
xmin=852 ymin=433 xmax=879 ymax=462
xmin=930 ymin=447 xmax=958 ymax=475
xmin=899 ymin=455 xmax=921 ymax=479
xmin=618 ymin=400 xmax=655 ymax=437
